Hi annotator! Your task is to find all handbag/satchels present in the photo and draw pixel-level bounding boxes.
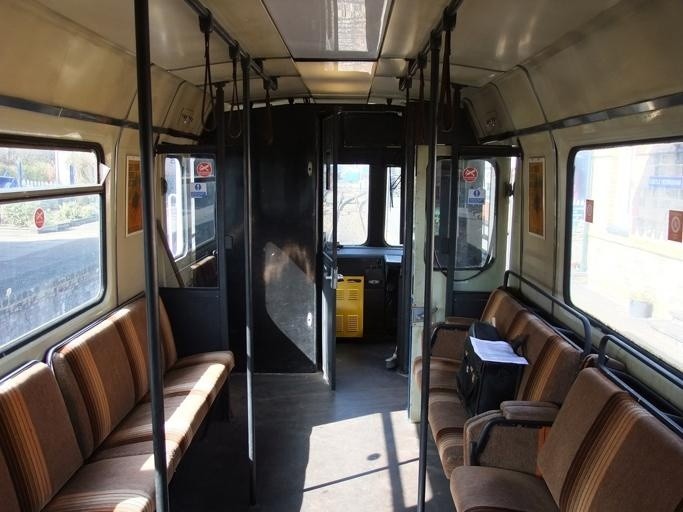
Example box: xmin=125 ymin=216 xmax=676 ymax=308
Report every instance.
xmin=455 ymin=320 xmax=529 ymax=421
